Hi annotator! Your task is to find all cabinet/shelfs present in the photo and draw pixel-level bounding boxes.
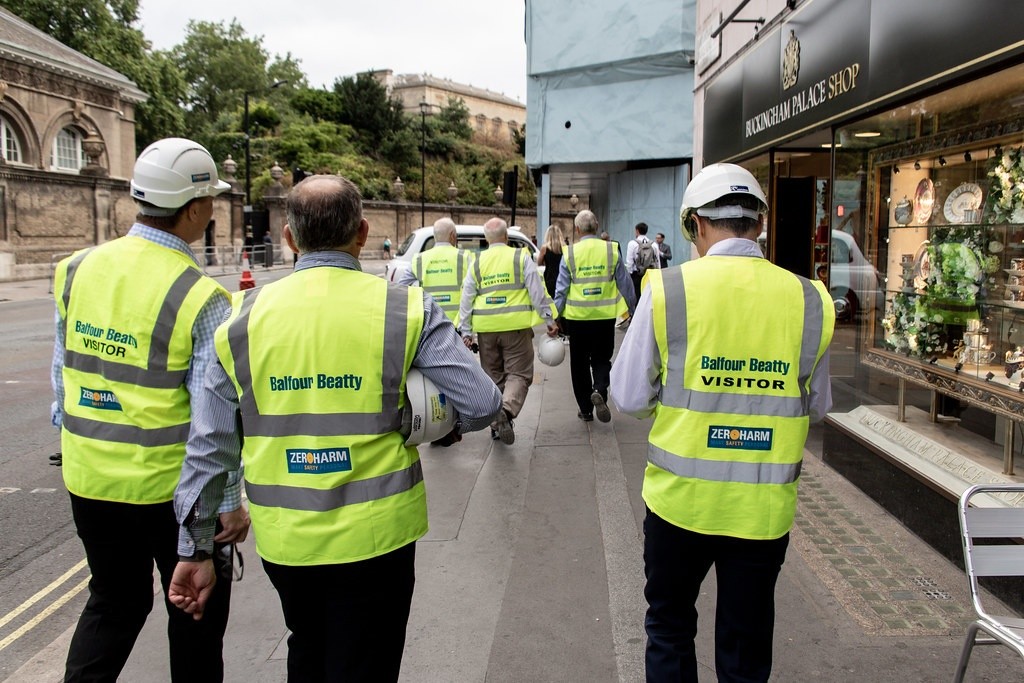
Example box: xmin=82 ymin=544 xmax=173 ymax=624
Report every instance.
xmin=887 ymin=221 xmax=1024 ymax=311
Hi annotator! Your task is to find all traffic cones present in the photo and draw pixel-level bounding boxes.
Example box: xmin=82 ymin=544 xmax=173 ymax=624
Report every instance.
xmin=239 ymin=251 xmax=256 ymax=290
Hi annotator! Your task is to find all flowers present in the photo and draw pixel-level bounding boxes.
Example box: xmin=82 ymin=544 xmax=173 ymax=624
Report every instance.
xmin=872 ymin=145 xmax=1024 ymax=362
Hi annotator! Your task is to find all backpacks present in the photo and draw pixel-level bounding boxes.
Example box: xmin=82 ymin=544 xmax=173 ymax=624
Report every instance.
xmin=633 ymin=238 xmax=659 ymax=275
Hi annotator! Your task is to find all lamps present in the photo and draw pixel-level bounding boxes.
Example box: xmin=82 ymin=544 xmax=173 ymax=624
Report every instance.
xmin=914 ymin=159 xmax=921 ymax=170
xmin=964 ymin=151 xmax=971 ymax=160
xmin=893 ymin=164 xmax=900 ymax=174
xmin=985 ymin=372 xmax=995 ymax=382
xmin=995 ymin=144 xmax=1003 ymax=156
xmin=955 ymin=363 xmax=964 ymax=373
xmin=1019 ymin=381 xmax=1024 ymax=391
xmin=930 ymin=356 xmax=938 ymax=365
xmin=939 ymin=155 xmax=946 ymax=167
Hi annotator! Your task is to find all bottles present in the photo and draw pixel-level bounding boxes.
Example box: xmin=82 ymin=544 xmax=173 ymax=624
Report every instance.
xmin=894 ymin=195 xmax=912 ymax=227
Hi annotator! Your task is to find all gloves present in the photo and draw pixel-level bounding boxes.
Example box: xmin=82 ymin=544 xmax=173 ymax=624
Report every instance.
xmin=431 ymin=428 xmax=461 ymax=447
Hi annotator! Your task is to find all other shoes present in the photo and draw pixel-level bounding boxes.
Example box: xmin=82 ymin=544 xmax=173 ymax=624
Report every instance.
xmin=578 ymin=409 xmax=593 ymax=422
xmin=591 ymin=390 xmax=611 ymax=422
xmin=491 ymin=429 xmax=500 ymax=440
xmin=496 ymin=407 xmax=515 ymax=445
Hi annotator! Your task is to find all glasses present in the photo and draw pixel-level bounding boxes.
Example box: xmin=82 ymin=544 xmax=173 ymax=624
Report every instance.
xmin=215 ymin=539 xmax=245 ymax=582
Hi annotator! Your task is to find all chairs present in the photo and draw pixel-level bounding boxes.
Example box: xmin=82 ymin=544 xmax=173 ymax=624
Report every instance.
xmin=953 ymin=482 xmax=1024 ymax=683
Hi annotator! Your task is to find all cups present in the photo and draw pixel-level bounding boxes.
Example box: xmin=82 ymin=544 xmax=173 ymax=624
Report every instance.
xmin=959 ymin=318 xmax=996 ymax=365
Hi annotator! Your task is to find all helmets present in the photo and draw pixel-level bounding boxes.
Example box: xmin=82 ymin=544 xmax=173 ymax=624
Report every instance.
xmin=398 ymin=366 xmax=462 ymax=447
xmin=679 ymin=162 xmax=769 ymax=242
xmin=537 ymin=333 xmax=565 ymax=366
xmin=615 ymin=314 xmax=632 ymax=334
xmin=130 ymin=137 xmax=231 ymax=208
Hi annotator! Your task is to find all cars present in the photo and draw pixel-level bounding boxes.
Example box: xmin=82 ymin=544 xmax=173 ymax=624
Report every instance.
xmin=759 ymin=228 xmax=884 ymax=324
xmin=383 ymin=225 xmax=547 ymax=293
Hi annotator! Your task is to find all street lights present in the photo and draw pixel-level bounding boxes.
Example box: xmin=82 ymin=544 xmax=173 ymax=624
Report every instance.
xmin=419 ymin=98 xmax=431 ymax=229
xmin=243 ymin=78 xmax=295 ymax=260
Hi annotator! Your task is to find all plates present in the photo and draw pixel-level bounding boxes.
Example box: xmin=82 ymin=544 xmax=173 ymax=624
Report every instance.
xmin=913 ymin=176 xmax=934 ymax=225
xmin=943 ymin=184 xmax=982 ymax=222
xmin=913 ymin=240 xmax=930 ymax=288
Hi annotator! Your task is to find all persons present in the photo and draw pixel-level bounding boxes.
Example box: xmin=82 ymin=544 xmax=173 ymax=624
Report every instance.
xmin=244 ymin=209 xmax=671 ymax=445
xmin=49 ymin=138 xmax=250 ymax=683
xmin=608 ymin=163 xmax=838 ymax=683
xmin=166 ymin=173 xmax=502 ymax=683
xmin=912 ymin=219 xmax=985 ymax=357
